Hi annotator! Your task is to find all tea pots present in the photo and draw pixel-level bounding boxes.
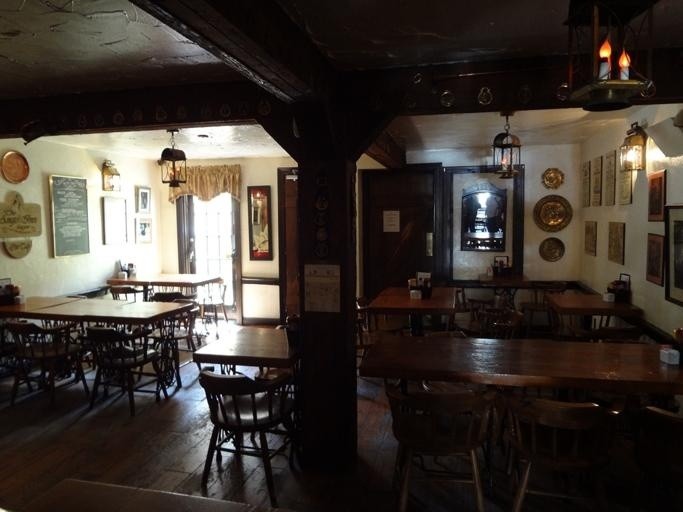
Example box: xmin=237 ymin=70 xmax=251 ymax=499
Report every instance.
xmin=607 ymin=279 xmax=628 ymax=301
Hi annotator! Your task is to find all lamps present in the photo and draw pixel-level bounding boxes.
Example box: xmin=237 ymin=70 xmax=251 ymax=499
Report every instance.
xmin=158 ymin=130 xmax=191 ymax=187
xmin=102 ymin=159 xmax=122 ymax=192
xmin=644 ymin=108 xmax=683 ymax=158
xmin=618 ymin=122 xmax=645 ymax=171
xmin=492 ymin=114 xmax=521 ymax=181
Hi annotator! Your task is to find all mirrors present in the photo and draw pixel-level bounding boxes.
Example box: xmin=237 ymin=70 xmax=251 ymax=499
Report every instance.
xmin=461 ymin=178 xmax=507 ymax=251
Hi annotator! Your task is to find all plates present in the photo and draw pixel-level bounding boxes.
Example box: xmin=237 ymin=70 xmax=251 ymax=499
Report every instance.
xmin=540 ymin=167 xmax=565 ymax=190
xmin=532 ymin=194 xmax=573 ymax=233
xmin=538 ymin=237 xmax=564 ymax=262
xmin=1 ymin=150 xmax=29 ymax=184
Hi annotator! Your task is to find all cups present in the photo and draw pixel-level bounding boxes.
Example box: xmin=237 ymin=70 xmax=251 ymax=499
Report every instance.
xmin=117 ymin=263 xmax=136 ymax=279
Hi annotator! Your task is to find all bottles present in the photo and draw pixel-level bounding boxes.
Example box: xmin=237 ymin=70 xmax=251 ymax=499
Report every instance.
xmin=407 ymin=279 xmax=416 ymax=291
xmin=486 ymin=259 xmax=504 ymax=278
xmin=0 ymin=283 xmax=21 ymax=304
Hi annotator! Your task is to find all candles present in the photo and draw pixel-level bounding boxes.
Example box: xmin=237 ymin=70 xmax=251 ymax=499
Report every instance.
xmin=597 ymin=39 xmax=611 ymax=81
xmin=618 ymin=47 xmax=631 ymax=79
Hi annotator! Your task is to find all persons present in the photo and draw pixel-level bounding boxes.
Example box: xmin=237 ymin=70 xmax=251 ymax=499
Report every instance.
xmin=467 ymin=193 xmax=481 ymax=233
xmin=485 ymin=194 xmax=500 ymax=240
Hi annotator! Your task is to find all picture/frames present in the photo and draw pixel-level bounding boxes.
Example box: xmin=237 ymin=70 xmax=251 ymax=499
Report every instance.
xmin=246 ymin=185 xmax=272 ymax=261
xmin=102 ymin=195 xmax=128 ymax=244
xmin=645 ymin=169 xmax=682 ymax=308
xmin=134 ymin=216 xmax=152 ymax=245
xmin=132 ymin=184 xmax=151 ymax=214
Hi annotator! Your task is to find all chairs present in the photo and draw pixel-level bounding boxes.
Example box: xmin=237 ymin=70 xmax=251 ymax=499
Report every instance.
xmin=497 ymin=391 xmax=605 ymax=511
xmin=199 ymin=366 xmax=295 ymax=509
xmin=1 ymin=273 xmax=230 ymax=421
xmin=633 ymin=405 xmax=682 ymax=512
xmin=355 ymin=273 xmax=681 ymax=458
xmin=384 ymin=379 xmax=486 ymax=511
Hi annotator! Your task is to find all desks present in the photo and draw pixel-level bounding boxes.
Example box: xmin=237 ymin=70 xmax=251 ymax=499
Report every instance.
xmin=193 ymin=325 xmax=293 ymax=458
